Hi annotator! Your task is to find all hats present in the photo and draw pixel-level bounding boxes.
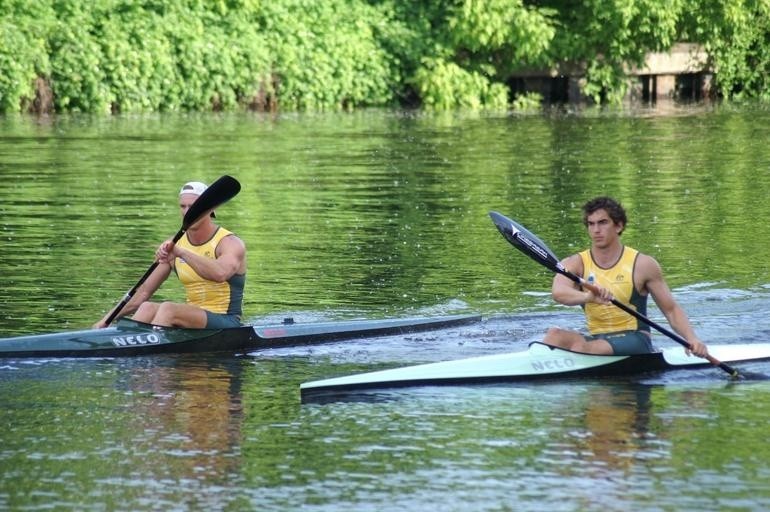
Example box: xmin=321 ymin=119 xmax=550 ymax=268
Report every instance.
xmin=177 ymin=182 xmax=218 ymax=219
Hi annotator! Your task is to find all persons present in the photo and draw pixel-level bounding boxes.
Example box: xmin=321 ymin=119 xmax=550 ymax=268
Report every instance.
xmin=543 ymin=197 xmax=707 ymax=359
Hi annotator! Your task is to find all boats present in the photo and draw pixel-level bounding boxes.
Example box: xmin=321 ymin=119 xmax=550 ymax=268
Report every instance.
xmin=1 ymin=310 xmax=483 ymax=359
xmin=299 ymin=342 xmax=770 ymax=404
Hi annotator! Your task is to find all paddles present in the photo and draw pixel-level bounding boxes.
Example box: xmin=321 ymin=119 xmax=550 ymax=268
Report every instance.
xmin=487 ymin=208 xmax=769 ymax=381
